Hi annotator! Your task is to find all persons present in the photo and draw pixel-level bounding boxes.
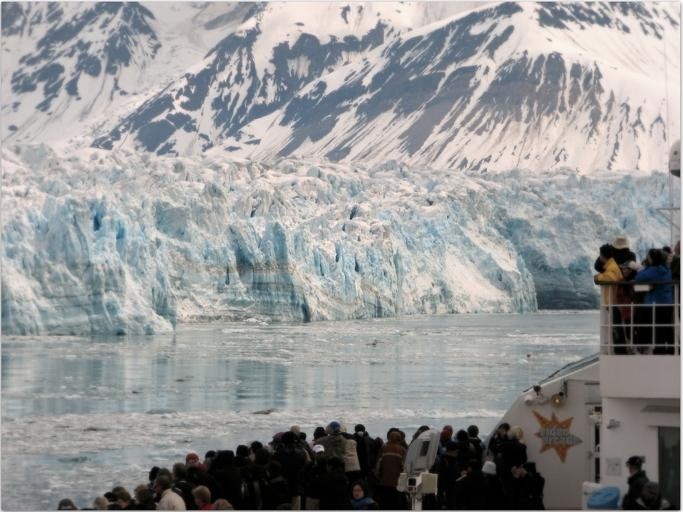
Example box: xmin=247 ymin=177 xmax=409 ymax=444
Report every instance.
xmin=593 ymin=232 xmax=680 ymax=356
xmin=54 ymin=420 xmax=545 ymax=511
xmin=634 ymin=481 xmax=671 ymax=509
xmin=621 ymin=455 xmax=649 ymax=501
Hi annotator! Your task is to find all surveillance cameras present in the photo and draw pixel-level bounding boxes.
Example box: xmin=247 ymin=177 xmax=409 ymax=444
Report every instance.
xmin=407 ymin=477 xmax=422 ymax=488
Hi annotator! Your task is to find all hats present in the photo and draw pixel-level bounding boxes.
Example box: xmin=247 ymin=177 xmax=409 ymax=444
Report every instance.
xmin=612 ymin=234 xmax=632 ymax=249
xmin=149 ymin=466 xmax=161 ymax=480
xmin=481 ymin=460 xmax=497 ymax=475
xmin=311 ymin=421 xmax=340 ymax=441
xmin=186 ymin=453 xmax=199 ymax=463
